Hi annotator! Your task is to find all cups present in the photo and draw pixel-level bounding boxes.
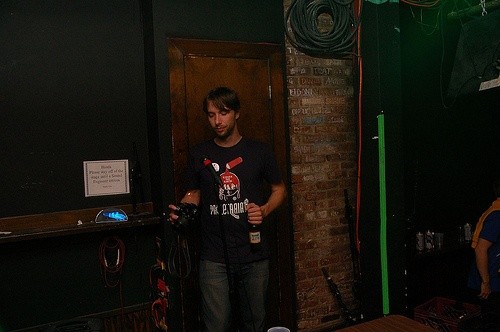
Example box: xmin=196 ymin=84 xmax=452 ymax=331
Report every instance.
xmin=267 ymin=326 xmax=289 ymax=332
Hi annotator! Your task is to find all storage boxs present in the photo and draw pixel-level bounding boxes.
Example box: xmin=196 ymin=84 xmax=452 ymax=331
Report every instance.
xmin=414 ymin=297 xmax=481 ymax=332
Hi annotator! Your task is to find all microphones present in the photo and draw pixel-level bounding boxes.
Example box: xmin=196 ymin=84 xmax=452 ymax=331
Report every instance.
xmin=200 ymin=154 xmax=223 ymax=187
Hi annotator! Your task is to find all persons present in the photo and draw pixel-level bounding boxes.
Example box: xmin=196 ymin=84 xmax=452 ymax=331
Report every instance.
xmin=168 ymin=86 xmax=288 ymax=332
xmin=471 ymin=199 xmax=500 ymax=332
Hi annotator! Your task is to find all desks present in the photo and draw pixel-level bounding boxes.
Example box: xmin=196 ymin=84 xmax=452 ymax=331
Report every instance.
xmin=333 ymin=315 xmax=440 ymax=332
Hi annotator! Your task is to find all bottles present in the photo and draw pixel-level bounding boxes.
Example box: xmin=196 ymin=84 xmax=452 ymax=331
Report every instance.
xmin=248 ymin=223 xmax=262 ymax=252
